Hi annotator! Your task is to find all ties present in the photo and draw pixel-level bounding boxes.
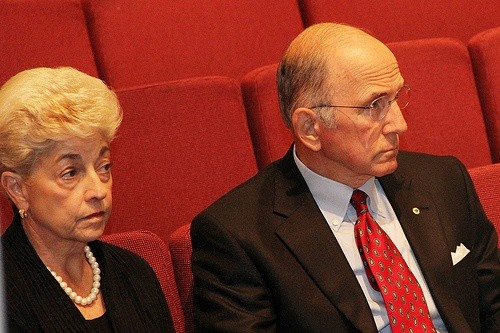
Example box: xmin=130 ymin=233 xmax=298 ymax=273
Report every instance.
xmin=349 ymin=187 xmax=436 ymax=333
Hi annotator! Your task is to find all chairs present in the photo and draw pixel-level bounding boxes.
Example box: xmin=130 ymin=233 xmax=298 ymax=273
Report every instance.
xmin=0 ymin=0 xmax=500 ymax=333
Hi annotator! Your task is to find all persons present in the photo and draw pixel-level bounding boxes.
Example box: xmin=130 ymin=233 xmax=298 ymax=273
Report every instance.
xmin=0 ymin=67 xmax=176 ymax=333
xmin=190 ymin=23 xmax=500 ymax=333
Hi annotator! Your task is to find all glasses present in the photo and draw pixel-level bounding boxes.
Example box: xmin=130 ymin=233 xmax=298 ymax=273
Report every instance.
xmin=309 ymin=85 xmax=411 ymax=120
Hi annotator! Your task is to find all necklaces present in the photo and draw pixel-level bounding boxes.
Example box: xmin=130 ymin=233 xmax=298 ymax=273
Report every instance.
xmin=47 ymin=246 xmax=100 ymax=305
